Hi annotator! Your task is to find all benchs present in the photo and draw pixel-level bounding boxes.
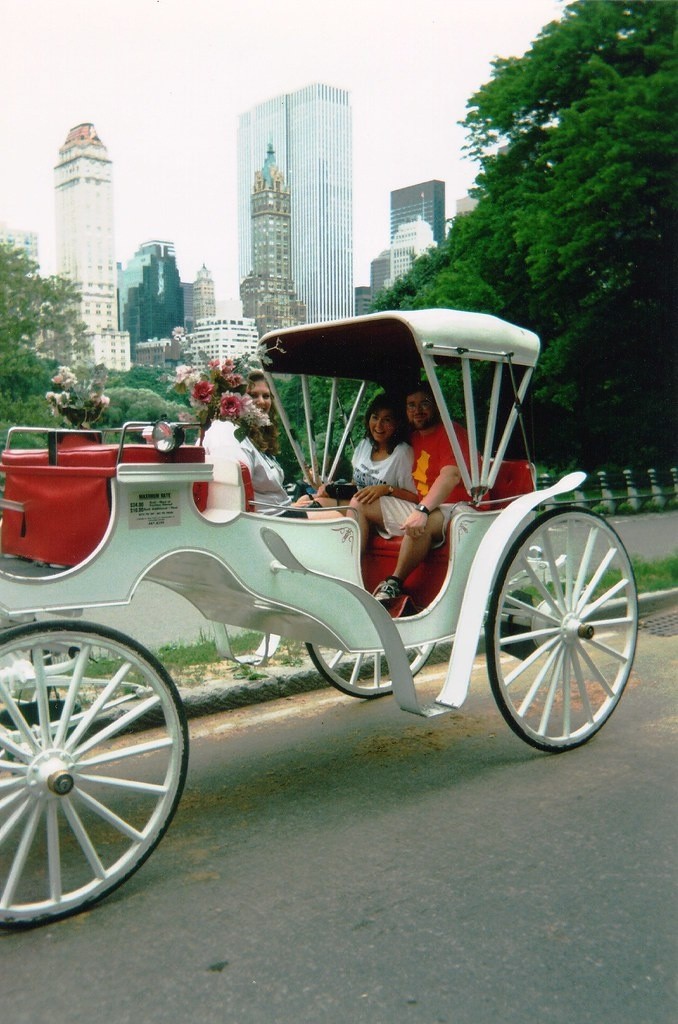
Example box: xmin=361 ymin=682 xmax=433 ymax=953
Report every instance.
xmin=360 ymin=458 xmax=536 ymax=607
xmin=0 ymin=419 xmax=207 ymax=566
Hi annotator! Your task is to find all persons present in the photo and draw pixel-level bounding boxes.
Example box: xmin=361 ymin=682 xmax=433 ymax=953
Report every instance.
xmin=293 ymin=393 xmax=418 ymax=515
xmin=345 ymin=384 xmax=492 ymax=607
xmin=293 ymin=463 xmax=324 ymax=502
xmin=192 ymin=370 xmax=346 ymax=521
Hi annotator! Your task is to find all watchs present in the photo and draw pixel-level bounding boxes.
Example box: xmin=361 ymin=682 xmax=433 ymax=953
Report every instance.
xmin=384 ymin=485 xmax=394 ymax=496
xmin=414 ymin=504 xmax=430 ymax=515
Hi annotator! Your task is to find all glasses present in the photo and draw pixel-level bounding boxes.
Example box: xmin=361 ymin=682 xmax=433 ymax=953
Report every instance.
xmin=406 ymin=401 xmax=435 ymax=410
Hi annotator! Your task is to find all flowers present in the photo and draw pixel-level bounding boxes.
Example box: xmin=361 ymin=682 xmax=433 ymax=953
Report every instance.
xmin=45 ymin=365 xmax=110 ymax=430
xmin=165 ymin=324 xmax=286 ymax=453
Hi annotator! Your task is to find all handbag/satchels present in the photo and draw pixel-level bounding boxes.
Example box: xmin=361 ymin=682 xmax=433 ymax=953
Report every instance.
xmin=325 ymin=484 xmax=358 ymax=506
xmin=306 ymin=501 xmax=322 ymax=508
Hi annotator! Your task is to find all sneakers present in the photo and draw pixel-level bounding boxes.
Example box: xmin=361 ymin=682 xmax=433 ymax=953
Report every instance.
xmin=372 ymin=580 xmax=401 ymax=603
